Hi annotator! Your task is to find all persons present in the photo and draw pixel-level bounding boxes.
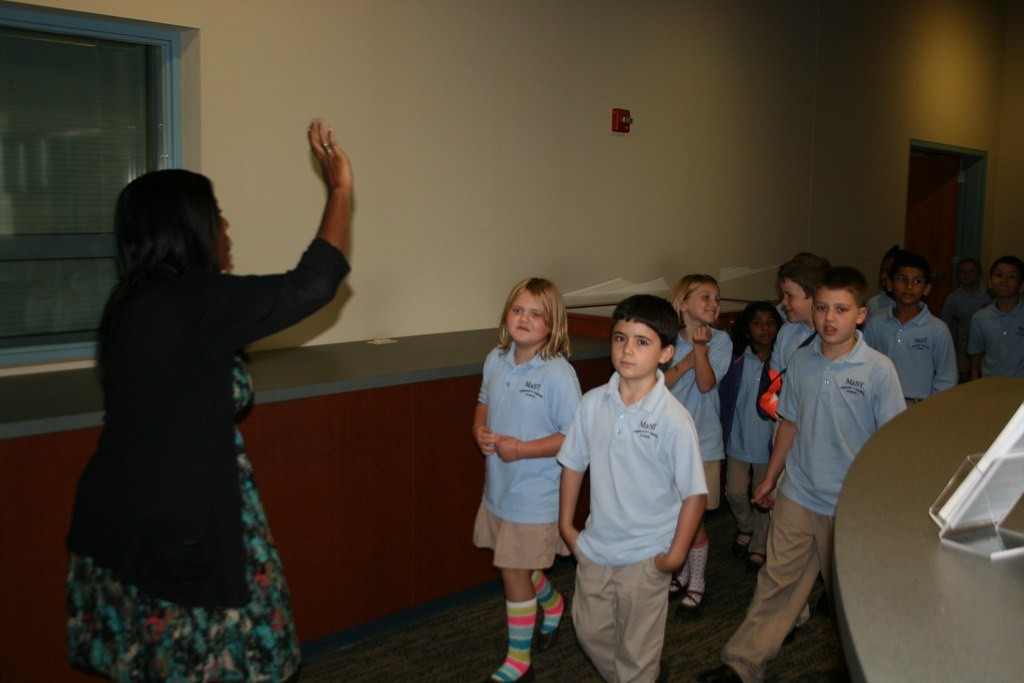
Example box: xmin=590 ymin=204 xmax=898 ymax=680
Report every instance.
xmin=966 ymin=255 xmax=1024 ymax=381
xmin=699 ymin=263 xmax=907 ymax=683
xmin=473 ymin=277 xmax=582 ymax=682
xmin=63 ymin=117 xmax=355 ymax=682
xmin=663 ymin=274 xmax=734 ymax=613
xmin=775 ymin=303 xmax=788 ymax=320
xmin=556 ymin=293 xmax=709 ymax=683
xmin=755 ymin=252 xmax=837 ymax=645
xmin=720 ymin=300 xmax=785 ymax=568
xmin=941 ymin=256 xmax=992 ymax=385
xmin=863 ymin=245 xmax=958 ymax=406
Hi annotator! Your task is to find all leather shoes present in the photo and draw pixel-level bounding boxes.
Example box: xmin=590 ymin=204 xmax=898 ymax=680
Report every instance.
xmin=483 ymin=661 xmax=534 ymax=683
xmin=536 ymin=593 xmax=569 ymax=651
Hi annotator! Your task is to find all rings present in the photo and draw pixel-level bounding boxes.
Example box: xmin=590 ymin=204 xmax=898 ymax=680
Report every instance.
xmin=323 ymin=144 xmax=331 ymax=153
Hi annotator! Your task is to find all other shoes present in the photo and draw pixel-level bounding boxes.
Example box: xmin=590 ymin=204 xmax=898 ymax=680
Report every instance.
xmin=676 ymin=590 xmax=707 ymax=618
xmin=666 ymin=575 xmax=688 ymax=602
xmin=655 ymin=659 xmax=670 ymax=683
xmin=699 ymin=664 xmax=743 ymax=683
xmin=783 ymin=628 xmax=801 ymax=645
xmin=817 ymin=593 xmax=829 ymax=615
xmin=733 ymin=527 xmax=753 ymax=559
xmin=748 ymin=551 xmax=766 ymax=580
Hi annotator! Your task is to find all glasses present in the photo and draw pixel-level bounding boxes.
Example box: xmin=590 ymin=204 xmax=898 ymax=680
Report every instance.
xmin=751 ymin=319 xmax=777 ymax=329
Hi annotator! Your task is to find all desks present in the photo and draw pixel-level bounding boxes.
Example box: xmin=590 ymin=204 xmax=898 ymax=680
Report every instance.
xmin=835 ymin=371 xmax=1024 ymax=682
